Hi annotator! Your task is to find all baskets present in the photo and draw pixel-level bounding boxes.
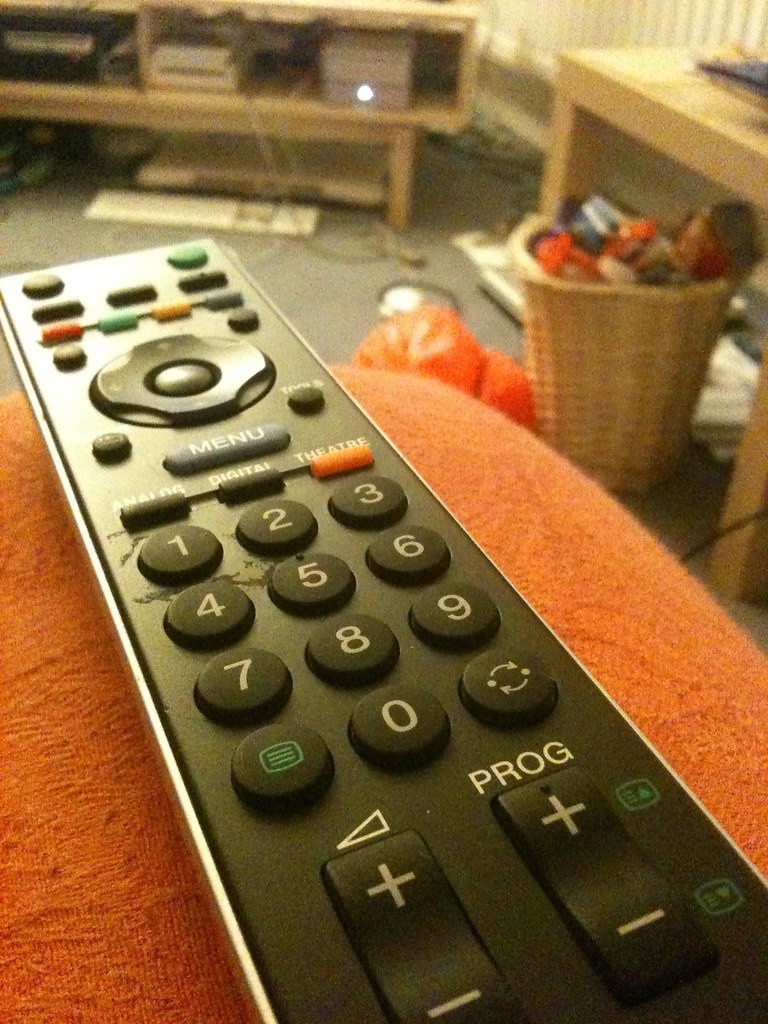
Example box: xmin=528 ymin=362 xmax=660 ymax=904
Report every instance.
xmin=512 ymin=214 xmax=735 ymax=496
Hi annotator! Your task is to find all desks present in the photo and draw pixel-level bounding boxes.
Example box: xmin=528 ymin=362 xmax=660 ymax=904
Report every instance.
xmin=536 ymin=48 xmax=768 ymax=607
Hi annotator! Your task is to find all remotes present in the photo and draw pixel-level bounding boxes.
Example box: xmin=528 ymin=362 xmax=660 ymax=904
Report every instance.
xmin=0 ymin=234 xmax=768 ymax=1024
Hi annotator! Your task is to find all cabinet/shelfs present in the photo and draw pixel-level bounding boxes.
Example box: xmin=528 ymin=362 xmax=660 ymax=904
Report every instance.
xmin=0 ymin=0 xmax=481 ymax=230
